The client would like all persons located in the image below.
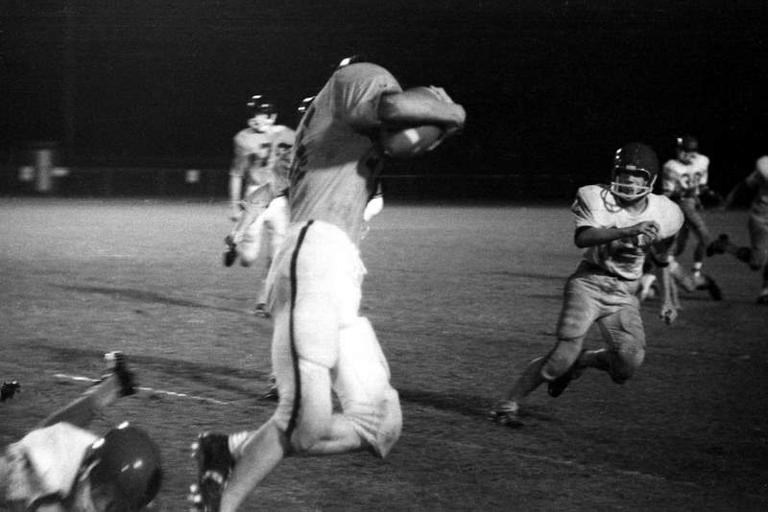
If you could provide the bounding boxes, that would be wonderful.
[641,134,724,307]
[0,349,165,512]
[221,94,299,316]
[490,143,686,429]
[188,53,467,511]
[704,149,767,305]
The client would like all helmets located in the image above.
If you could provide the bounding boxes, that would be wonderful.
[607,140,658,209]
[244,92,280,132]
[675,136,697,157]
[88,420,166,511]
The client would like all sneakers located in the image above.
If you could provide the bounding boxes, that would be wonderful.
[224,235,240,267]
[105,348,139,397]
[667,232,732,302]
[183,432,238,512]
[548,349,588,400]
[490,398,523,427]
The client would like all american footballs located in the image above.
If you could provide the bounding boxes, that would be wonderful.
[380,85,447,158]
